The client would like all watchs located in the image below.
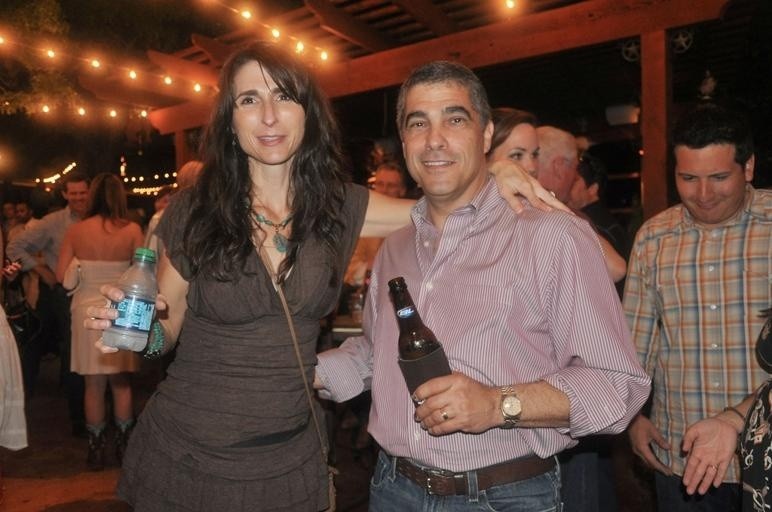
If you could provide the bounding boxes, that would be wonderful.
[138,321,162,361]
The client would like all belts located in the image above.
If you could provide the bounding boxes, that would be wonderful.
[380,446,556,498]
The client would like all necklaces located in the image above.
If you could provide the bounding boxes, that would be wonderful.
[245,204,293,252]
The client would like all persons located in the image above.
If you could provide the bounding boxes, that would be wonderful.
[619,105,772,512]
[313,57,650,512]
[1,159,205,470]
[85,41,577,512]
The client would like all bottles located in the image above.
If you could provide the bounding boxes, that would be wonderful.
[350,268,371,311]
[387,277,451,410]
[101,246,158,354]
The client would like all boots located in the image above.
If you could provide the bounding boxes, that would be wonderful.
[83,421,110,470]
[113,418,136,462]
